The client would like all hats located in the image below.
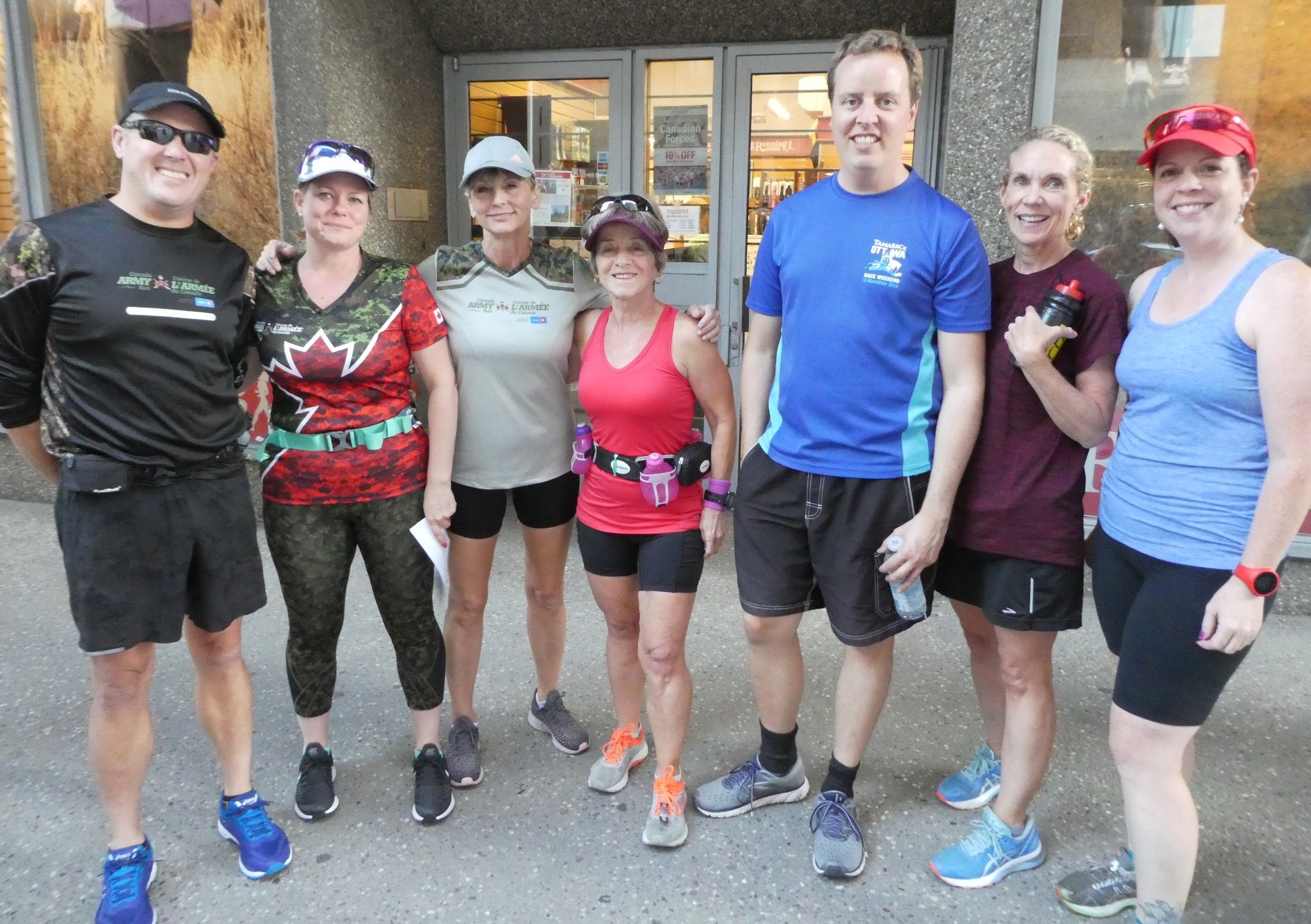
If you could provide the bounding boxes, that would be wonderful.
[119,81,227,139]
[459,136,536,187]
[585,208,663,253]
[298,143,378,192]
[1137,103,1256,178]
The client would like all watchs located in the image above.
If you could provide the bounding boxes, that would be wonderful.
[1229,560,1280,598]
[700,488,737,512]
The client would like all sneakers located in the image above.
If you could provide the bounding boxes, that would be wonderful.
[294,742,339,820]
[411,743,455,823]
[95,833,158,924]
[528,687,590,754]
[929,806,1045,889]
[810,787,868,877]
[587,720,648,792]
[217,786,292,879]
[935,744,1002,810]
[445,716,483,786]
[691,753,809,817]
[642,766,688,846]
[1056,845,1136,917]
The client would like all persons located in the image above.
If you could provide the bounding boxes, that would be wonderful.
[683,31,991,886]
[927,125,1131,894]
[231,137,456,826]
[0,80,295,924]
[1047,103,1310,924]
[73,0,193,128]
[562,195,734,846]
[250,132,724,790]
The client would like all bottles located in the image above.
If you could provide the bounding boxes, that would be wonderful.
[570,422,592,475]
[689,428,702,443]
[640,453,680,505]
[1008,279,1084,369]
[883,536,927,620]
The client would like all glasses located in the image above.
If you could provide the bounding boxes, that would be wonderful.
[584,195,658,222]
[1144,106,1258,155]
[117,118,220,155]
[299,141,375,181]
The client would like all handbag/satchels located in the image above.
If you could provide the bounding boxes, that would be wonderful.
[674,442,712,486]
